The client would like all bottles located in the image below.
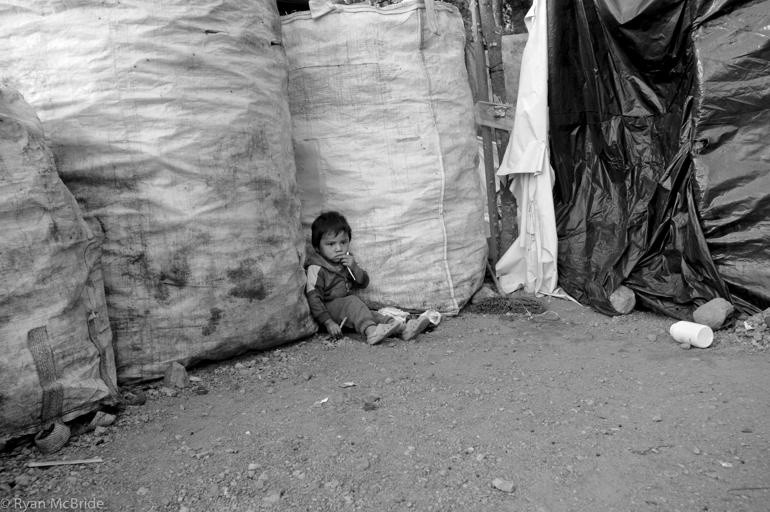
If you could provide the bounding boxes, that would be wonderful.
[670,320,714,349]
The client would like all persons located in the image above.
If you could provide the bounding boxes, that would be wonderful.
[301,211,432,347]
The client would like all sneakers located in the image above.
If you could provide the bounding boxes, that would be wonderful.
[401,316,430,342]
[366,321,400,345]
